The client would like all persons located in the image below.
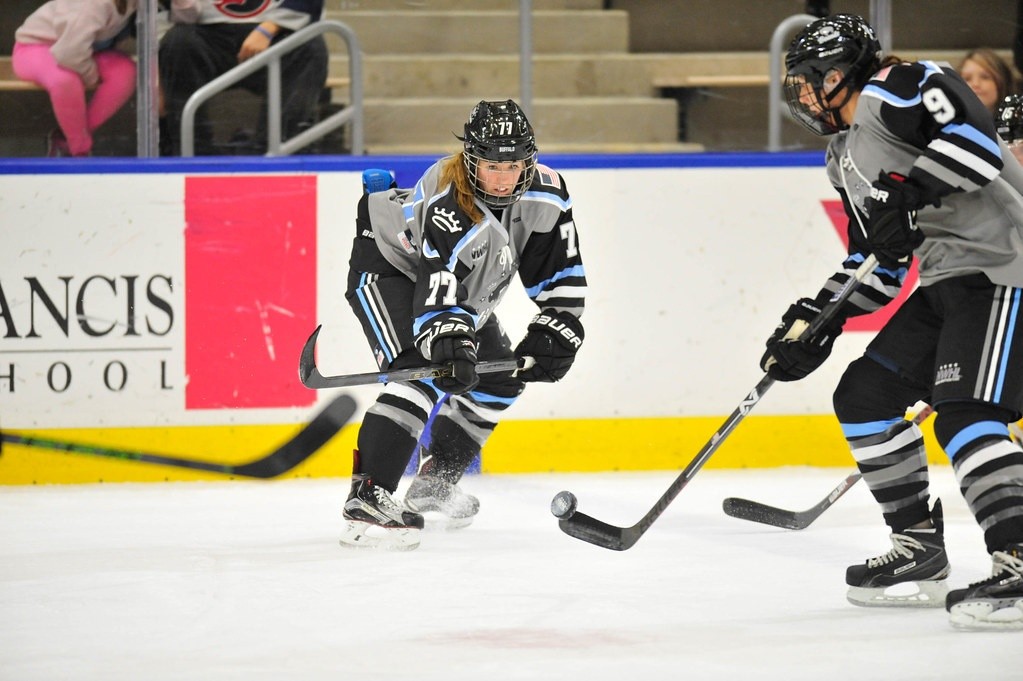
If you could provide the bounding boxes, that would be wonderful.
[994,94,1023,144]
[12,0,137,157]
[759,14,1023,632]
[338,100,587,552]
[158,0,328,156]
[957,49,1012,127]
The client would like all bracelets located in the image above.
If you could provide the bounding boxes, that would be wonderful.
[256,25,272,40]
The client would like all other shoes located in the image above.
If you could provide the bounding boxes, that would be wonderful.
[78,148,93,157]
[47,128,71,157]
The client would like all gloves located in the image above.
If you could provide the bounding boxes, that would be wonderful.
[513,308,585,382]
[759,298,846,381]
[866,166,926,271]
[430,334,480,396]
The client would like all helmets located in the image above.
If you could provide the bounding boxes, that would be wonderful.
[782,13,882,137]
[462,99,538,210]
[994,93,1023,147]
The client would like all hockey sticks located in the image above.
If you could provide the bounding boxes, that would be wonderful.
[559,252,880,552]
[3,392,357,480]
[299,325,538,390]
[722,405,935,531]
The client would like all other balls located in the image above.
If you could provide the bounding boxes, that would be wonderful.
[551,490,578,521]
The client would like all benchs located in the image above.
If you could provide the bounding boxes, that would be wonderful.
[0,53,352,152]
[647,49,1023,143]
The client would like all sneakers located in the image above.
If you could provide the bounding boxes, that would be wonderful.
[946,542,1023,632]
[846,497,951,604]
[403,478,480,530]
[339,447,424,546]
